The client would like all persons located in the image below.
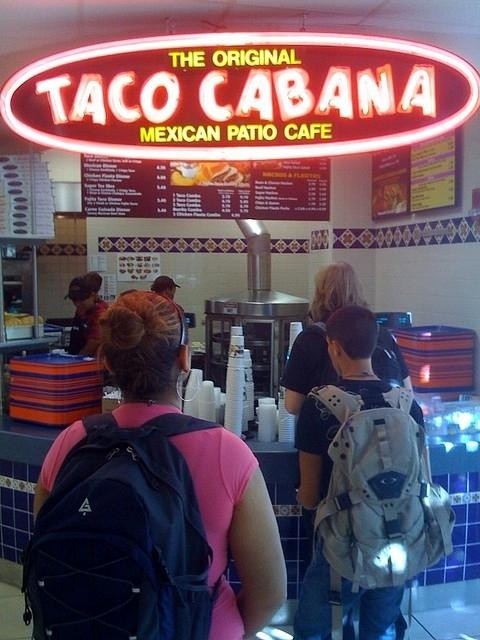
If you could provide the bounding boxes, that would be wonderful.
[63,271,111,356]
[33,291,287,640]
[151,274,181,301]
[277,261,413,529]
[290,305,427,640]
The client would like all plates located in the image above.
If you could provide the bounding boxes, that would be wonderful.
[394,325,477,392]
[8,354,104,429]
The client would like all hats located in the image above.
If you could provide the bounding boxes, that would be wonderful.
[151,276,181,291]
[64,277,94,301]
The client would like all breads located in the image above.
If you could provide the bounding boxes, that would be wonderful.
[3,311,43,327]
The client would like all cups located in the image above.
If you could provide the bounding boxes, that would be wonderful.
[278,382,296,444]
[288,320,302,357]
[182,321,254,444]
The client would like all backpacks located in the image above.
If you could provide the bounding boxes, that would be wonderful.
[308,384,456,593]
[22,411,228,639]
